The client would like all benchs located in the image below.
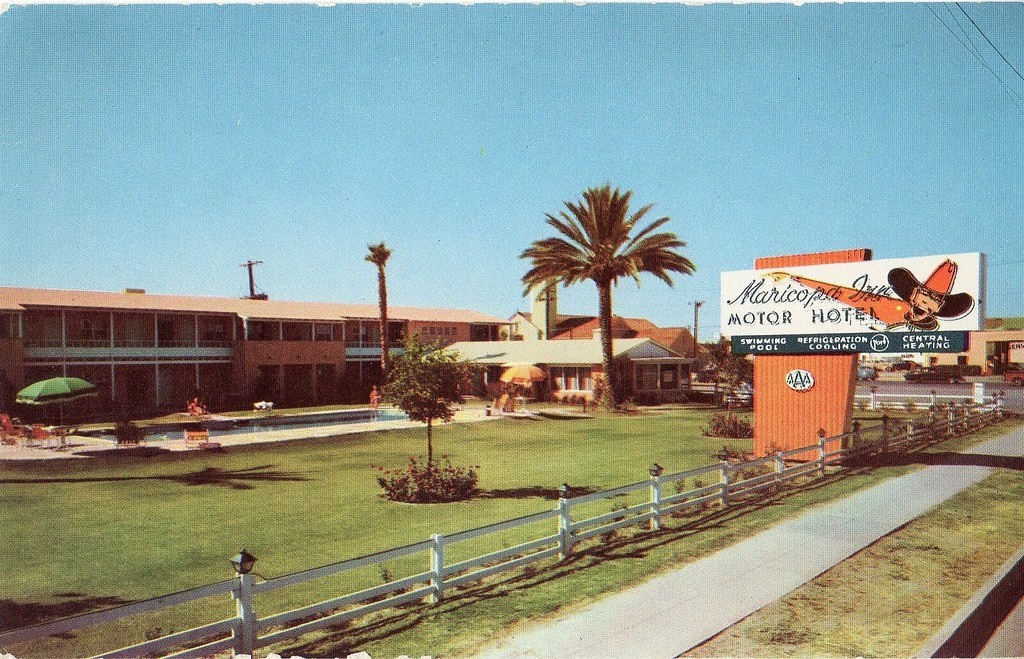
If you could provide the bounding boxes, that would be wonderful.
[183,429,210,449]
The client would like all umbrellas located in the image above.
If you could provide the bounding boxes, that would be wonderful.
[500,364,549,400]
[16,375,98,449]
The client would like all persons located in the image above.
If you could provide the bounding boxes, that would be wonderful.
[188,397,212,420]
[368,384,383,424]
[495,389,522,416]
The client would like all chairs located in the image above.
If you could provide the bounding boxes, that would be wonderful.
[1,413,51,449]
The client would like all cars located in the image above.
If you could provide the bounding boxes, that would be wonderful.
[856,359,1024,387]
